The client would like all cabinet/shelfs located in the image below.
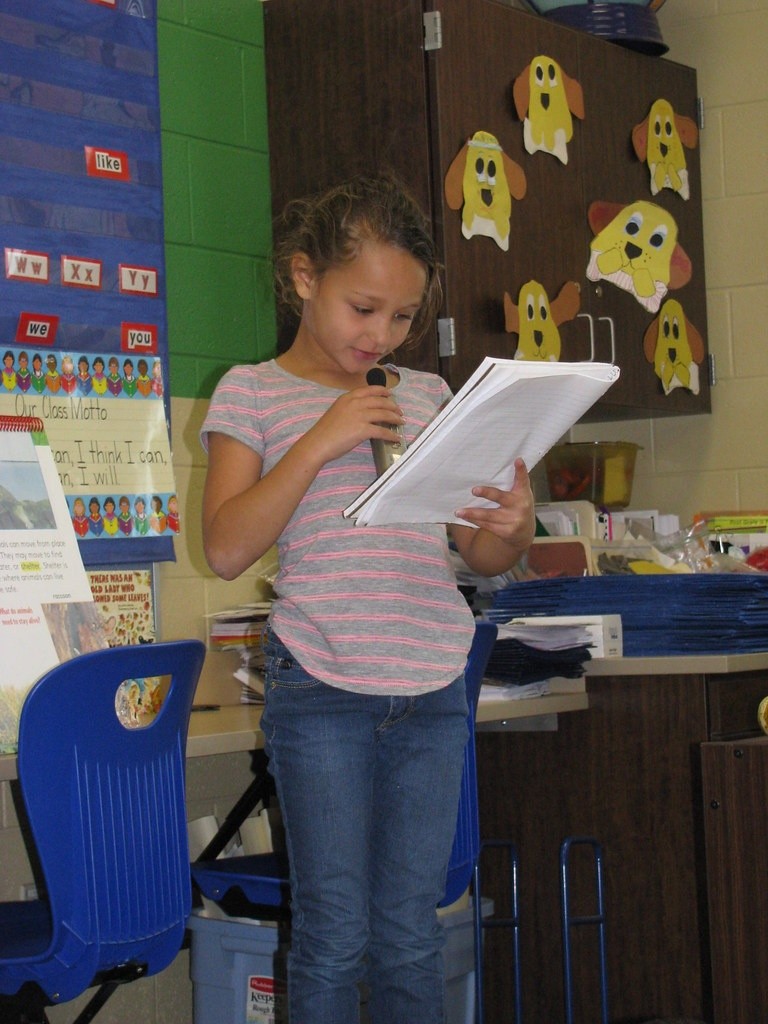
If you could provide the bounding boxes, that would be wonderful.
[263,0,715,425]
[475,653,768,1024]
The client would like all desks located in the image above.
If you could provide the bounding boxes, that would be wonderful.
[0,691,591,1024]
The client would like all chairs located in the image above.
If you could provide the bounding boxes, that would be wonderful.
[189,622,499,922]
[0,637,207,1024]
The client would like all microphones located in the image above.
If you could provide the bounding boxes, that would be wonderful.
[366,368,407,477]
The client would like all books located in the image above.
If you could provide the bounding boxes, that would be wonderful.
[208,355,766,706]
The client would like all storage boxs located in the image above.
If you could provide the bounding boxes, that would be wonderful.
[542,440,645,508]
[512,614,622,660]
[184,897,494,1024]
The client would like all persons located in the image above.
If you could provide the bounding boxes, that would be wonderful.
[198,168,540,1024]
